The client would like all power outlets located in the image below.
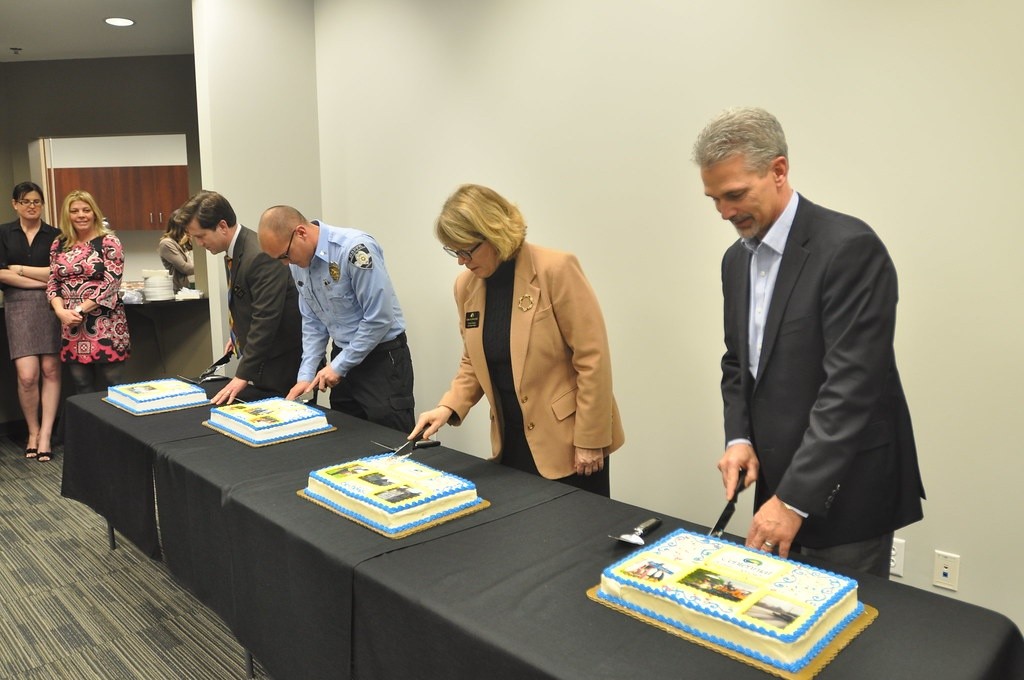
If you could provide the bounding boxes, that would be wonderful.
[889,538,905,578]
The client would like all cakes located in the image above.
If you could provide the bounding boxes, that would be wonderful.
[208,395,334,445]
[106,377,211,414]
[305,452,482,533]
[596,528,864,673]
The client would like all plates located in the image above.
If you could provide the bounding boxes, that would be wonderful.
[144,276,174,301]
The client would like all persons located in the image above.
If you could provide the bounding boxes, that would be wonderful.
[158,209,196,293]
[174,189,327,404]
[46,191,131,394]
[0,182,62,462]
[408,185,625,497]
[694,108,926,579]
[258,206,417,435]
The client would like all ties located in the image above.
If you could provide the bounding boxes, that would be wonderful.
[224,254,243,360]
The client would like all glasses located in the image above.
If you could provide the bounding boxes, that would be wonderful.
[272,230,296,261]
[15,198,44,207]
[442,237,486,261]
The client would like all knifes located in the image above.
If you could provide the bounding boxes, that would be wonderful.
[707,470,746,539]
[389,424,431,459]
[198,350,234,378]
[295,384,319,399]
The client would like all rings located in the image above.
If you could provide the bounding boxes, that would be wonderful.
[764,541,774,549]
[227,391,231,394]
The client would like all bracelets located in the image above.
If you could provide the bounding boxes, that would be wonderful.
[20,265,23,276]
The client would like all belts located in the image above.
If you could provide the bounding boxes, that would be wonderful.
[332,332,407,351]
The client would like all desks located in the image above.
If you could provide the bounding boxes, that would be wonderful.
[57,372,1023,680]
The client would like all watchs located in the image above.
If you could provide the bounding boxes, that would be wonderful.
[74,305,85,315]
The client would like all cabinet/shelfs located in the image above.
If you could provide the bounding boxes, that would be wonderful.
[27,137,189,231]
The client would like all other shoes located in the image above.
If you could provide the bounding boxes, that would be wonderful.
[25,448,52,462]
